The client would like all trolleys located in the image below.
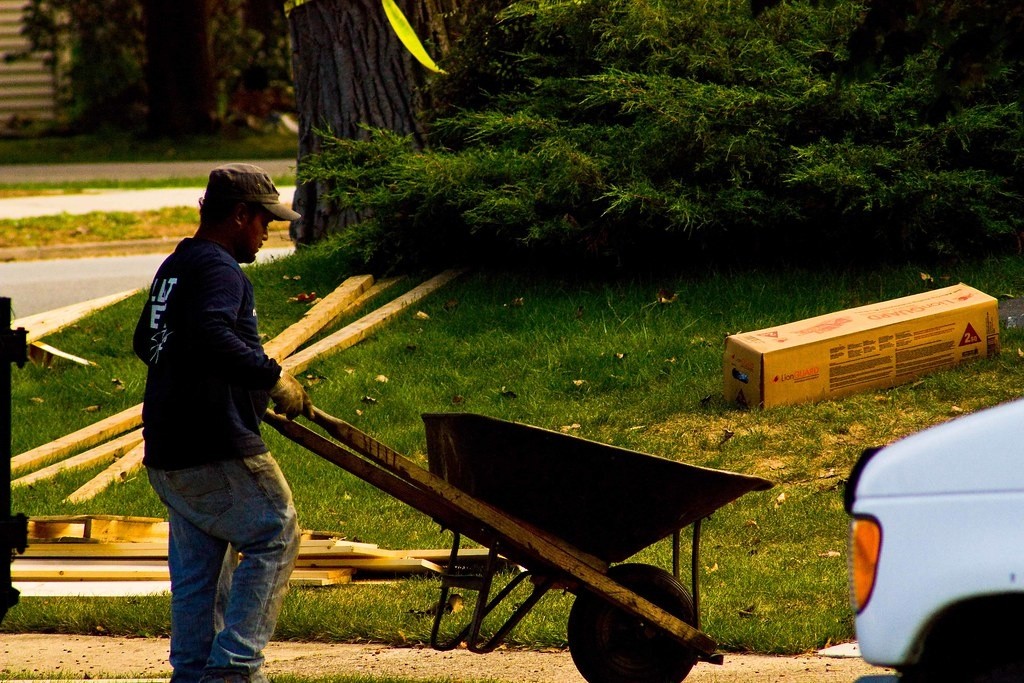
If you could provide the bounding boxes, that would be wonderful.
[261,404,778,683]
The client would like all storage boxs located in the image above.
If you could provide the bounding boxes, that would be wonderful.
[722,281,1001,412]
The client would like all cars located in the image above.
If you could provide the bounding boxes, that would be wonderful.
[842,399,1024,683]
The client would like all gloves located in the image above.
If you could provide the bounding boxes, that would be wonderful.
[269,371,315,421]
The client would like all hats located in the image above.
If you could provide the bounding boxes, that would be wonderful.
[207,161,301,221]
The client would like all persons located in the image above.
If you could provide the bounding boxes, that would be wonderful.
[131,163,315,683]
